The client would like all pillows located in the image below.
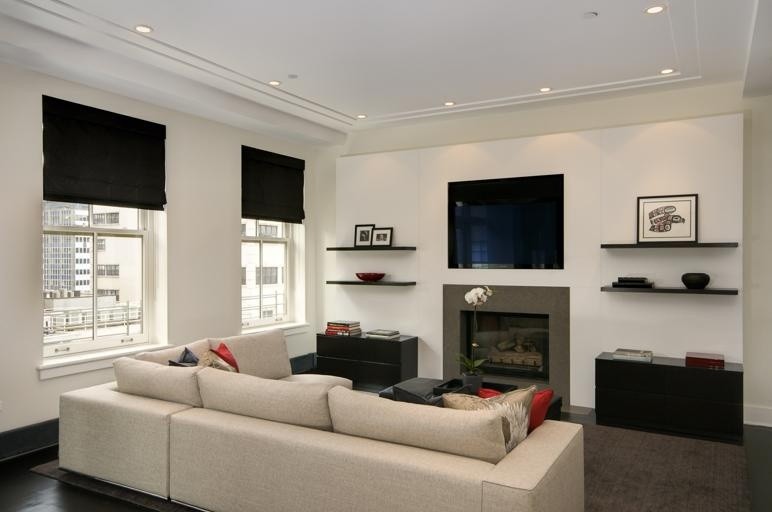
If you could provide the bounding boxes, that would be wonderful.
[195,350,238,373]
[166,345,201,368]
[207,341,240,373]
[392,384,444,408]
[544,393,563,423]
[379,389,392,399]
[477,386,555,434]
[440,383,538,456]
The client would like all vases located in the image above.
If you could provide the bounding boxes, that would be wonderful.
[458,369,484,394]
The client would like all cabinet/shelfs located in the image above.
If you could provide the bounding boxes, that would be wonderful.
[326,245,416,286]
[599,242,741,295]
[593,350,746,448]
[313,328,419,383]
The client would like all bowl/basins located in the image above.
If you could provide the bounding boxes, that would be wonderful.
[681,272,710,289]
[355,272,385,281]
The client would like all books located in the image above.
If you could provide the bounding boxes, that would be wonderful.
[612,348,653,362]
[612,277,655,288]
[325,320,402,340]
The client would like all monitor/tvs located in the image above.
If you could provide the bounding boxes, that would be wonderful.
[448,174,564,269]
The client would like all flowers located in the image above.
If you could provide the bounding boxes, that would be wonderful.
[450,284,497,377]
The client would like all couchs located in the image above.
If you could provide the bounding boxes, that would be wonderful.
[53,326,590,512]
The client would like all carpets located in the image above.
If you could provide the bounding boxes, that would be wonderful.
[30,409,755,512]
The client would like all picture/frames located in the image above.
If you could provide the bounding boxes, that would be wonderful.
[637,194,698,241]
[354,224,375,247]
[370,227,393,248]
